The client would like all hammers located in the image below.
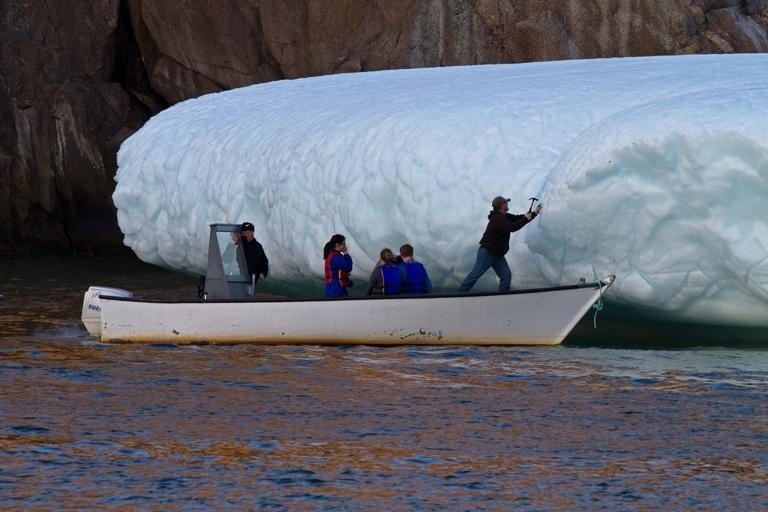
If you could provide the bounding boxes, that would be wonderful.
[527,197,539,213]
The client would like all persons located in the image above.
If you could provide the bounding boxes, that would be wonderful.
[324,235,352,297]
[222,231,248,275]
[400,244,432,294]
[241,223,268,285]
[458,195,542,292]
[364,249,409,297]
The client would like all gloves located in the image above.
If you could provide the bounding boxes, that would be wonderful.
[524,212,532,221]
[533,203,542,216]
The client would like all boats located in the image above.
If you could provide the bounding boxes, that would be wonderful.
[81,274,618,347]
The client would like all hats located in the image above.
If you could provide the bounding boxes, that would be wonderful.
[492,196,510,209]
[241,222,254,231]
